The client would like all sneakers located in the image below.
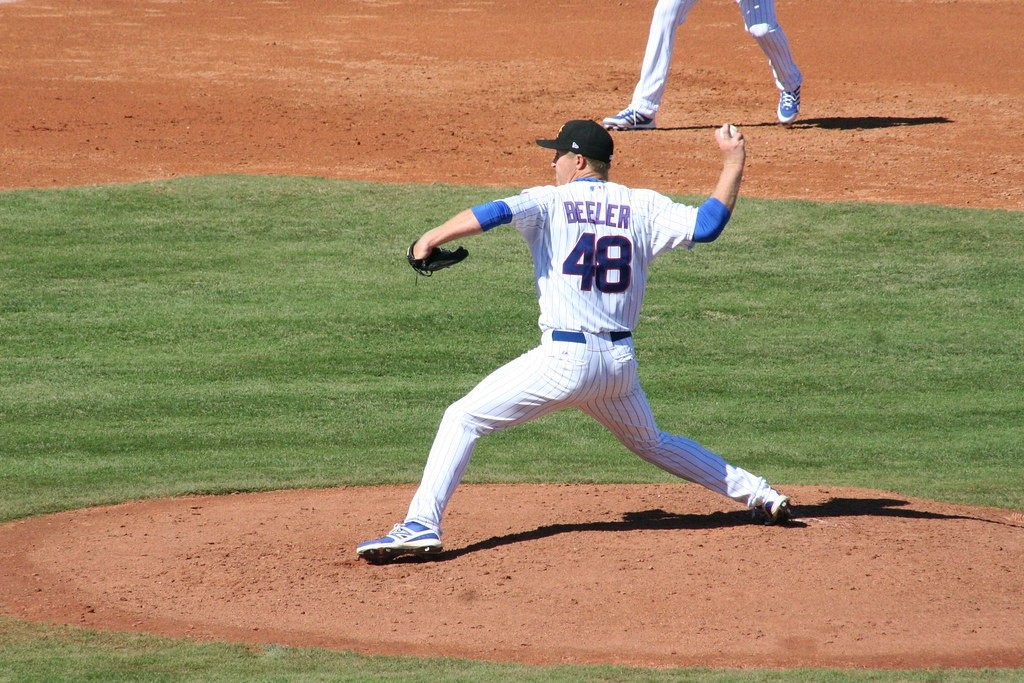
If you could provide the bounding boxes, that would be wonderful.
[603,107,656,130]
[356,522,442,563]
[752,488,791,524]
[777,85,801,125]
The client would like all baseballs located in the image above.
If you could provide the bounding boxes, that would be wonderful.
[719,124,738,138]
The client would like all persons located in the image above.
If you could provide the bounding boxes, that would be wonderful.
[356,120,795,562]
[603,0,803,129]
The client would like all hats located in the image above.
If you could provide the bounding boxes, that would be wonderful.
[536,119,614,164]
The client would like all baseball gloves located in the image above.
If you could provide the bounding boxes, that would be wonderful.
[405,240,469,272]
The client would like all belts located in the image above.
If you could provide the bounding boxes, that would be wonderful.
[552,330,632,344]
